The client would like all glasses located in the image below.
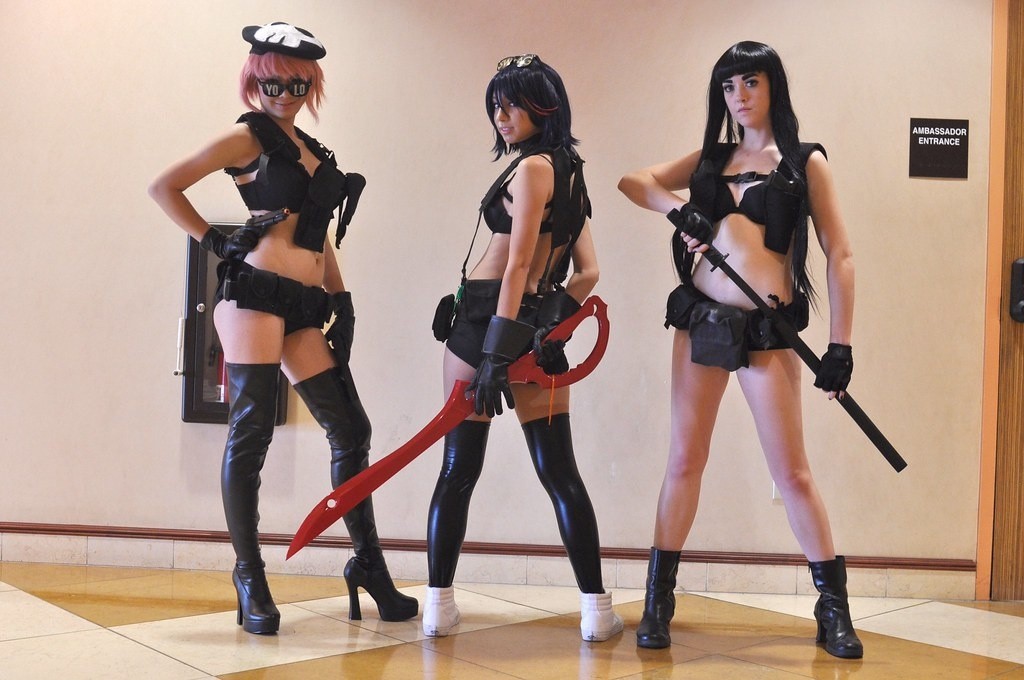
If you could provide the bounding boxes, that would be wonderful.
[496,53,536,71]
[256,78,312,97]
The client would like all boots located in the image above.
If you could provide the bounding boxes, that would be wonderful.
[291,365,419,621]
[635,547,682,648]
[807,554,863,658]
[220,363,281,634]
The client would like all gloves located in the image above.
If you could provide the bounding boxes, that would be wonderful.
[198,226,259,259]
[533,289,583,375]
[464,313,538,419]
[323,291,355,371]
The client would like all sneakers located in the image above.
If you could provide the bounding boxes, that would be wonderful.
[421,584,462,637]
[580,590,624,642]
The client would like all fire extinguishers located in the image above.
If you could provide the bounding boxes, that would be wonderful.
[207,340,229,404]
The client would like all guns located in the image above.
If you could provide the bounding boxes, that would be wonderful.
[765,169,805,199]
[234,207,290,260]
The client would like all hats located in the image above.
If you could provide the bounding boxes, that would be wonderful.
[241,22,327,60]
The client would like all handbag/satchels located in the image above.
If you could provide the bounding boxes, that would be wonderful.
[688,299,748,372]
[464,279,502,324]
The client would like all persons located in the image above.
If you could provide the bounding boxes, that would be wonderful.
[618,41,865,659]
[147,21,419,633]
[423,57,624,643]
